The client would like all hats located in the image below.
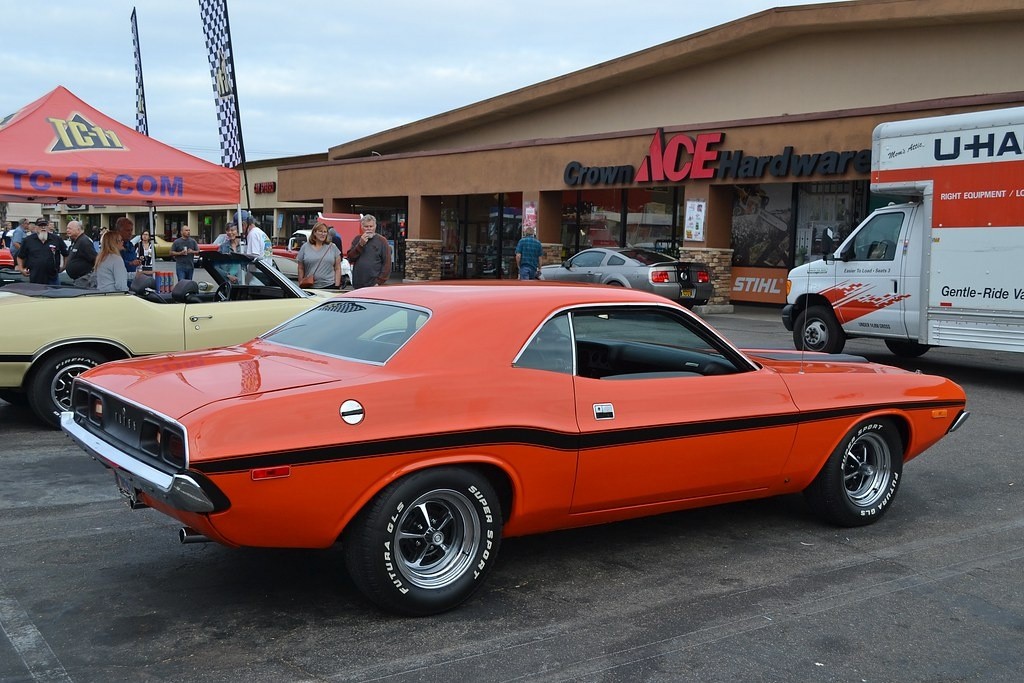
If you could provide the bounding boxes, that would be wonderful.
[231,211,251,228]
[37,218,48,226]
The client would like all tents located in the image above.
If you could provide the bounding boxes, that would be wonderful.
[0,84,245,254]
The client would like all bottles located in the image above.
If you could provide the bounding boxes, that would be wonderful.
[153,270,174,293]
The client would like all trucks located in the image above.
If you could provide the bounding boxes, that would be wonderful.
[780,106,1024,380]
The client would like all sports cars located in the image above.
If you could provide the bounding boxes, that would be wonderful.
[0,249,359,431]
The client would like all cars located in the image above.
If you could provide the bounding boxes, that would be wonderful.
[55,276,975,608]
[1,244,75,286]
[537,244,717,314]
[188,232,355,288]
[128,233,181,262]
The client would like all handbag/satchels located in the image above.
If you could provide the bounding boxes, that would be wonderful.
[299,275,315,289]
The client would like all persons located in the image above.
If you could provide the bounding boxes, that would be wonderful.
[91,225,111,247]
[229,210,274,288]
[0,218,61,271]
[326,225,343,263]
[346,213,392,290]
[515,227,543,281]
[88,231,130,293]
[169,225,199,282]
[14,217,69,285]
[115,217,144,279]
[134,229,154,278]
[213,223,243,286]
[64,221,98,289]
[294,222,343,289]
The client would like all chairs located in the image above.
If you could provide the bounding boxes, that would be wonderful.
[130,270,202,304]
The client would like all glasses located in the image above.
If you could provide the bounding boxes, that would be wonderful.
[116,239,123,243]
